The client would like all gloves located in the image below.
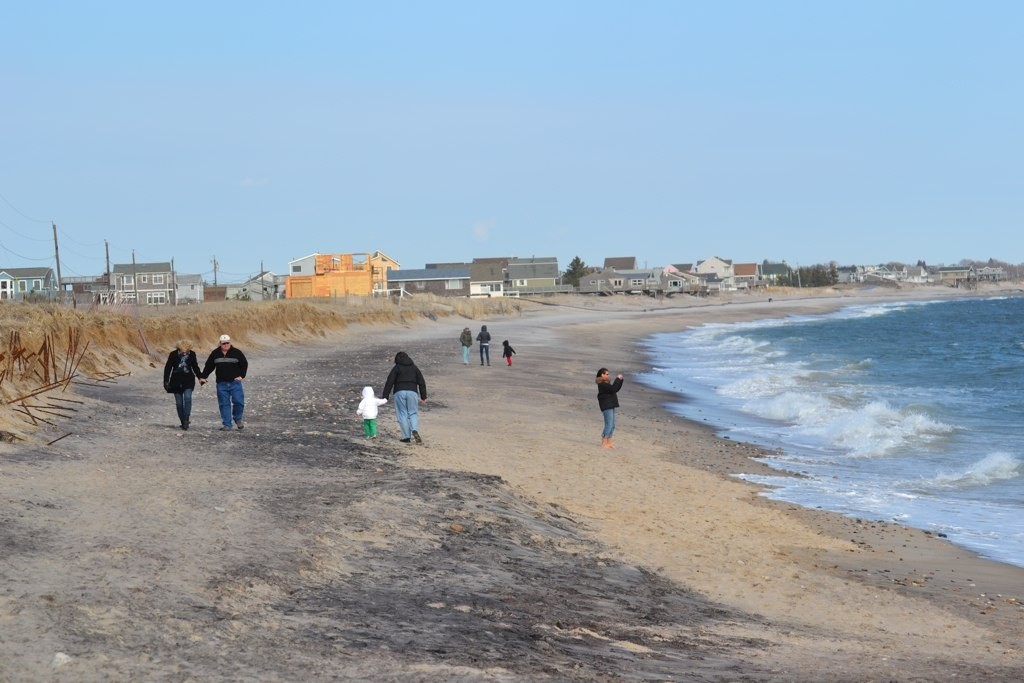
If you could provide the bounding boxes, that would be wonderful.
[164,381,169,391]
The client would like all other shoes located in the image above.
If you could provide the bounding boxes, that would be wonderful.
[237,422,244,429]
[181,421,190,430]
[412,429,421,443]
[221,425,231,431]
[371,434,376,439]
[366,436,370,440]
[400,438,410,442]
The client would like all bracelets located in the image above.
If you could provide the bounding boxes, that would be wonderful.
[239,376,242,380]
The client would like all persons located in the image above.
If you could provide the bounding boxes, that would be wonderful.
[475,325,491,366]
[594,367,624,449]
[502,339,516,366]
[355,386,388,439]
[163,339,208,430]
[201,334,249,430]
[382,351,427,444]
[460,326,473,365]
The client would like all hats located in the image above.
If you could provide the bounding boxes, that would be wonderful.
[219,335,230,343]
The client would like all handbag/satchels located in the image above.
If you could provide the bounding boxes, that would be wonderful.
[168,375,187,393]
